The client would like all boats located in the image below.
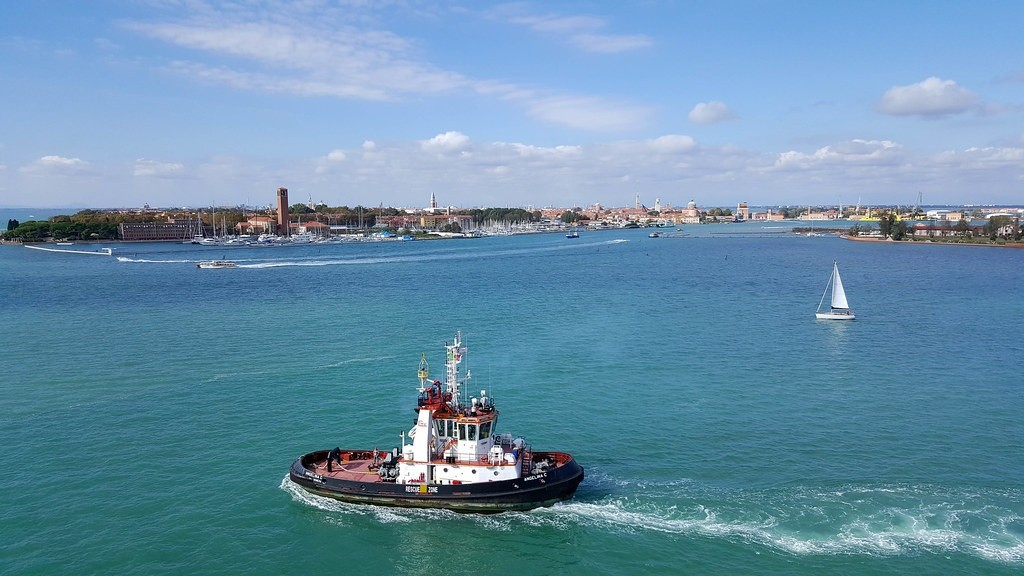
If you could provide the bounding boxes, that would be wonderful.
[284,328,587,518]
[183,205,689,240]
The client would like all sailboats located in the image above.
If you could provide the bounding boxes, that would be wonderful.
[814,260,855,320]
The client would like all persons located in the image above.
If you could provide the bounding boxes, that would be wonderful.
[373,447,380,463]
